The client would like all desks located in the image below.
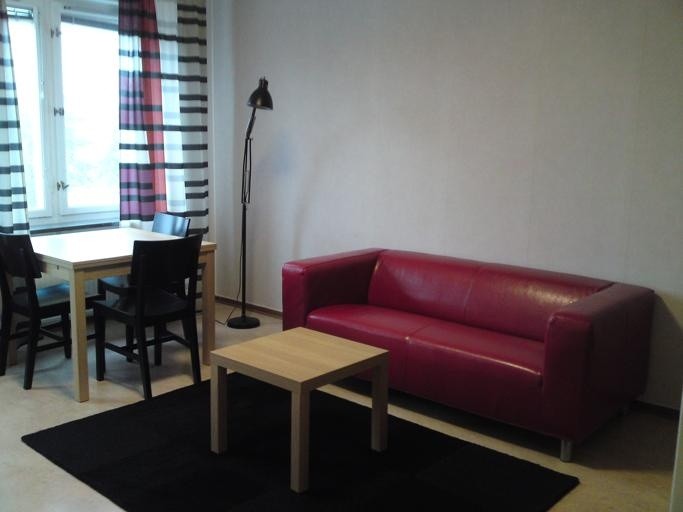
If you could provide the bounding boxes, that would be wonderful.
[202,324,390,495]
[25,233,217,403]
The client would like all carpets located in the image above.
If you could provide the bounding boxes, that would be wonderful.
[22,369,579,512]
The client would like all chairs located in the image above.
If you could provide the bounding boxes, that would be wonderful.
[92,210,193,368]
[91,226,212,400]
[0,230,107,390]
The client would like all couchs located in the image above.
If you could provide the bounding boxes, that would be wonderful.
[278,244,653,459]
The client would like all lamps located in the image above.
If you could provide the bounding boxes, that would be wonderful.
[227,74,275,328]
